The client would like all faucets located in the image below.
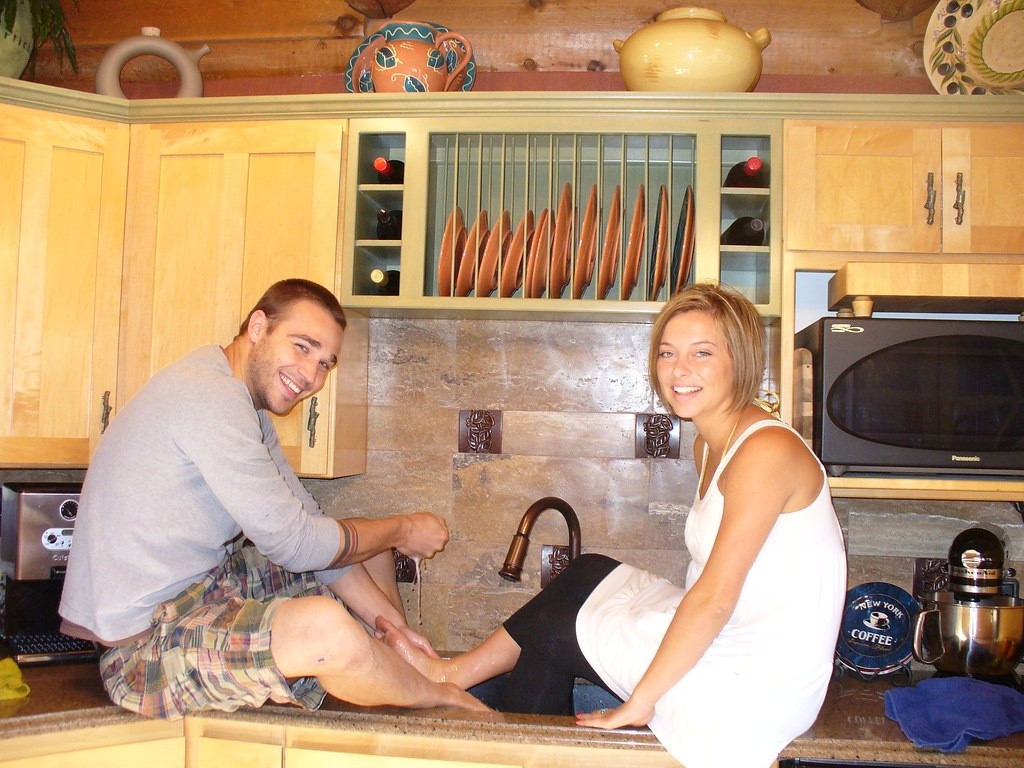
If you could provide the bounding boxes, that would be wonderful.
[499,496,581,583]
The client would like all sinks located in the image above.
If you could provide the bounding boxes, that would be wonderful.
[299,661,671,726]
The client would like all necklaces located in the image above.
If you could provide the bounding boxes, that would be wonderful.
[698,407,744,498]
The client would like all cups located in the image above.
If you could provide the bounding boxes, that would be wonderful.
[870,612,889,626]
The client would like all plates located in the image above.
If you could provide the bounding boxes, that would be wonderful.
[622,184,645,301]
[650,185,667,301]
[834,582,921,675]
[438,207,467,296]
[923,0,1024,95]
[478,210,513,297]
[500,210,535,298]
[863,619,890,630]
[550,184,578,299]
[345,21,476,93]
[597,185,626,300]
[457,210,490,297]
[670,185,695,299]
[574,184,602,300]
[525,208,547,299]
[531,209,556,299]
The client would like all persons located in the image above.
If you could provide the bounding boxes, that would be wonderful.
[374,281,846,767]
[60,279,504,713]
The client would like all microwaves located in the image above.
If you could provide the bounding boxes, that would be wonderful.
[794,317,1024,477]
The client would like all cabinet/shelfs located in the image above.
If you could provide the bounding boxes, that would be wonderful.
[1,76,1024,502]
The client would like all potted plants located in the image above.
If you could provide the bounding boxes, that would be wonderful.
[0,0,82,79]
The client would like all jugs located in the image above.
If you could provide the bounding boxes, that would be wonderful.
[372,20,472,92]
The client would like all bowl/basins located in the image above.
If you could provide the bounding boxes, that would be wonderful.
[911,591,1024,678]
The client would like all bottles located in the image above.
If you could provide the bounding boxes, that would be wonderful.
[723,157,764,188]
[374,157,404,184]
[721,217,764,246]
[377,208,402,240]
[370,269,400,296]
